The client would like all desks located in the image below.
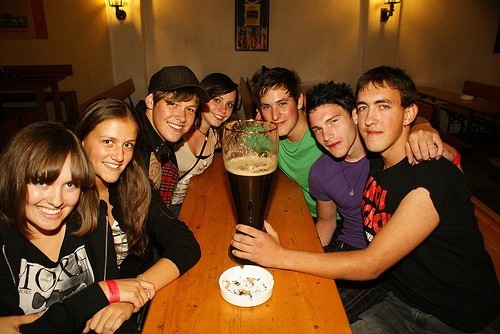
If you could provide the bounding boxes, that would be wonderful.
[416,86,500,140]
[139,153,352,334]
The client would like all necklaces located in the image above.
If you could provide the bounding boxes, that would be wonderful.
[340,157,367,196]
[198,129,208,141]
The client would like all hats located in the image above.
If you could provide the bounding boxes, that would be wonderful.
[148,66,210,104]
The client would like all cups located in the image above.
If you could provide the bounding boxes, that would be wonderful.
[222,118,279,267]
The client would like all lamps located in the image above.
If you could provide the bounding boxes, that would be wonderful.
[381,0,400,23]
[108,0,127,20]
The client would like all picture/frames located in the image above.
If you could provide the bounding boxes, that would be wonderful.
[235,0,270,51]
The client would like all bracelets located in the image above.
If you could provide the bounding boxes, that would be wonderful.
[104,279,120,303]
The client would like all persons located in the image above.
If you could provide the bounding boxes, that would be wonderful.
[0,99,201,334]
[133,65,210,213]
[230,66,500,334]
[251,66,343,252]
[172,73,239,219]
[307,80,444,301]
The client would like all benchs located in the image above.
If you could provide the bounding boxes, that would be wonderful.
[446,80,500,135]
[416,98,461,148]
